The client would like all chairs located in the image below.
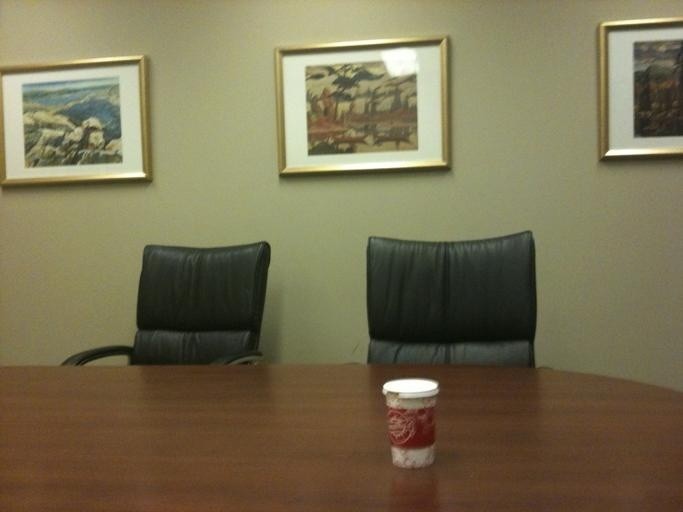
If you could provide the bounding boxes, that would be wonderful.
[360,230,536,369]
[63,243,276,368]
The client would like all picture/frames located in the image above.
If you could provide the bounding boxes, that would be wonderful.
[273,35,451,178]
[0,55,150,187]
[594,15,683,162]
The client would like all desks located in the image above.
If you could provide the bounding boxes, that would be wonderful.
[0,360,681,512]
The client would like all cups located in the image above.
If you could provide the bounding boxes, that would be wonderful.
[381,377,440,469]
